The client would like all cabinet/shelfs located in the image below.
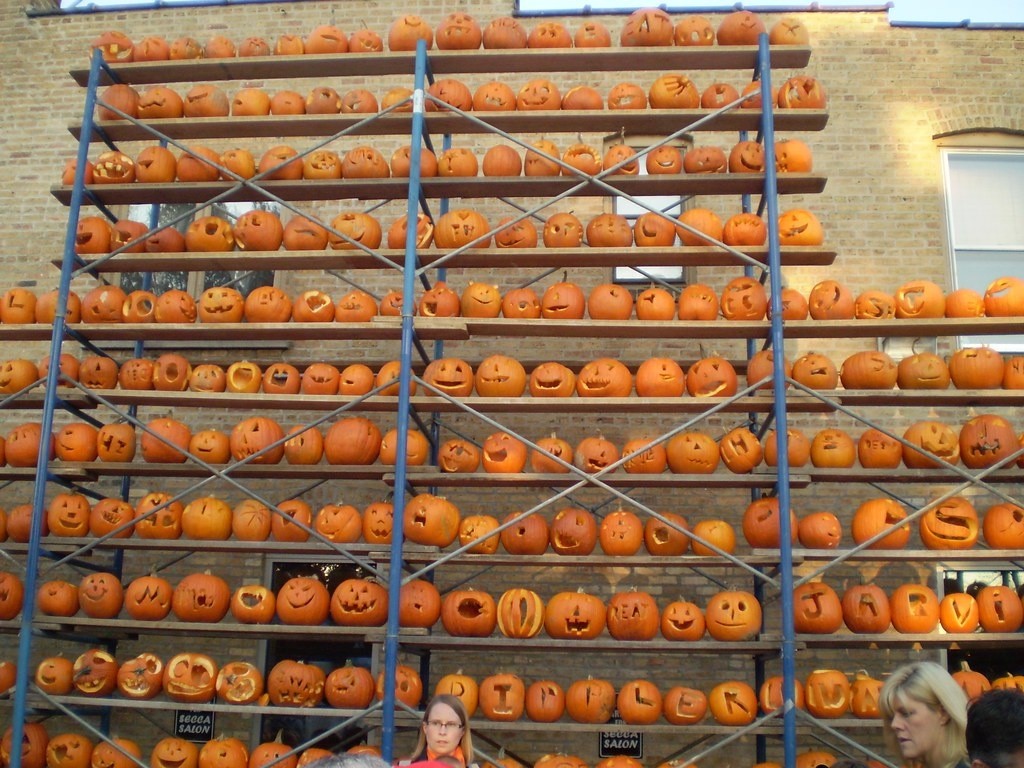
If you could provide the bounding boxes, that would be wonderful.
[0,32,1024,768]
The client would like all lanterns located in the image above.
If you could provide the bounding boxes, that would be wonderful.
[55,128,819,187]
[0,415,1023,472]
[1,648,1024,724]
[868,757,925,767]
[67,205,825,255]
[1,494,1023,556]
[80,6,810,60]
[485,747,838,768]
[0,343,1024,398]
[0,573,1024,642]
[97,72,827,121]
[1,274,1023,324]
[0,719,382,766]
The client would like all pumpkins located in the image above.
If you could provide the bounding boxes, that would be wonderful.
[482,144,522,178]
[98,84,139,121]
[676,208,723,246]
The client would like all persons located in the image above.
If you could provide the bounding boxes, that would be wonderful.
[964,686,1024,768]
[295,754,394,768]
[878,661,971,767]
[397,695,483,768]
[435,756,463,768]
[827,759,870,768]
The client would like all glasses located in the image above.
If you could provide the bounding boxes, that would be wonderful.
[428,720,465,729]
